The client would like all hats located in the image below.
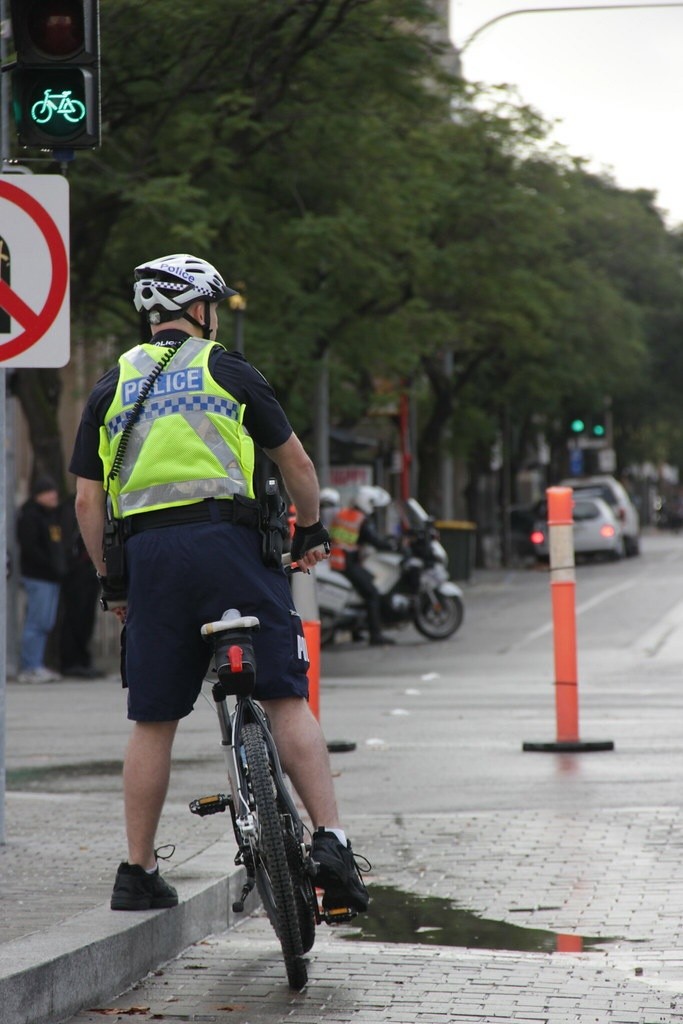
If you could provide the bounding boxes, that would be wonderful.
[30,472,57,494]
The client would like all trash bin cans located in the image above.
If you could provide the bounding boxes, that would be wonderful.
[434,520,479,580]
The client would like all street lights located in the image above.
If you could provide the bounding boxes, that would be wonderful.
[229,281,247,355]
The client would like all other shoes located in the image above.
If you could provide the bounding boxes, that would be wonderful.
[17,666,61,682]
[368,630,397,645]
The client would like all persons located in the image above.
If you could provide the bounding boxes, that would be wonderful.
[66,253,371,914]
[14,473,109,684]
[327,484,397,647]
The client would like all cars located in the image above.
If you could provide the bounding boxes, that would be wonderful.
[530,496,626,563]
[559,474,640,556]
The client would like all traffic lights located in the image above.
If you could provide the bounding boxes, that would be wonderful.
[588,418,606,438]
[568,416,588,435]
[7,0,102,151]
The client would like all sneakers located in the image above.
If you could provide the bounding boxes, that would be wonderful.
[110,848,179,910]
[309,826,369,913]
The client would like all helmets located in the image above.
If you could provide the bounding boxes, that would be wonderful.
[133,253,238,325]
[354,484,392,515]
[319,487,341,506]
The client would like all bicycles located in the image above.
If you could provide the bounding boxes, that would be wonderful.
[99,541,359,992]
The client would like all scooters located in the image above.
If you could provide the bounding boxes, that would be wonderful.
[314,498,464,650]
